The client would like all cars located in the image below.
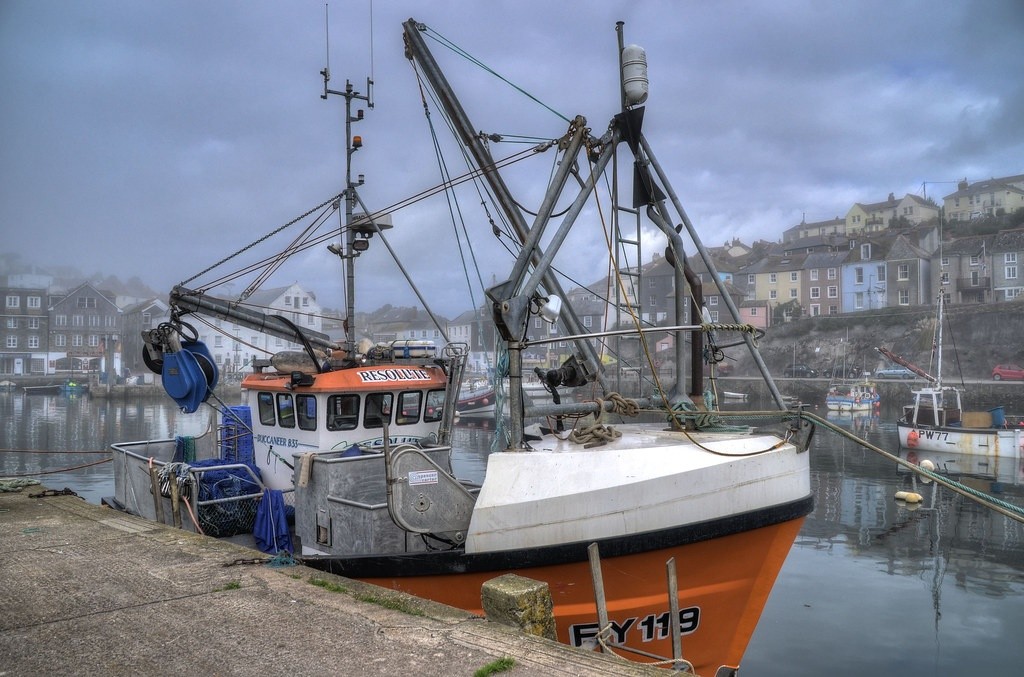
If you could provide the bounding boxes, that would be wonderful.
[822,364,861,380]
[874,363,914,380]
[990,360,1022,381]
[784,363,817,378]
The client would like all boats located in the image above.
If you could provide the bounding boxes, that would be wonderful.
[894,274,1023,457]
[825,323,880,411]
[104,0,816,671]
